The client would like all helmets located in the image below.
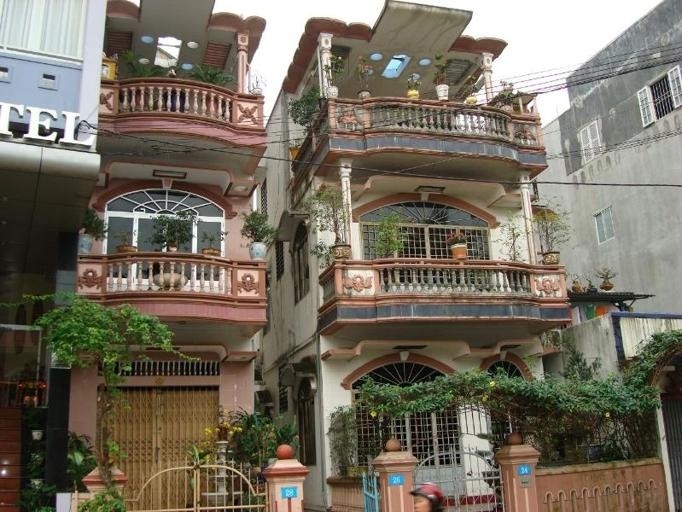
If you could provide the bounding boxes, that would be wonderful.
[407,484,444,507]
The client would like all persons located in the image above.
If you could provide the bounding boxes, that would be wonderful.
[409,484,445,512]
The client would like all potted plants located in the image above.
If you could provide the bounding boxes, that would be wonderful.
[370,203,416,292]
[19,405,51,490]
[323,51,517,115]
[76,203,280,262]
[305,183,353,259]
[120,51,238,121]
[570,265,617,293]
[492,188,573,292]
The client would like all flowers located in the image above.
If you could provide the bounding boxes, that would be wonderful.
[445,231,467,245]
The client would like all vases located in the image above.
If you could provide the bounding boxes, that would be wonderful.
[450,244,467,260]
[289,146,299,159]
[577,441,604,464]
